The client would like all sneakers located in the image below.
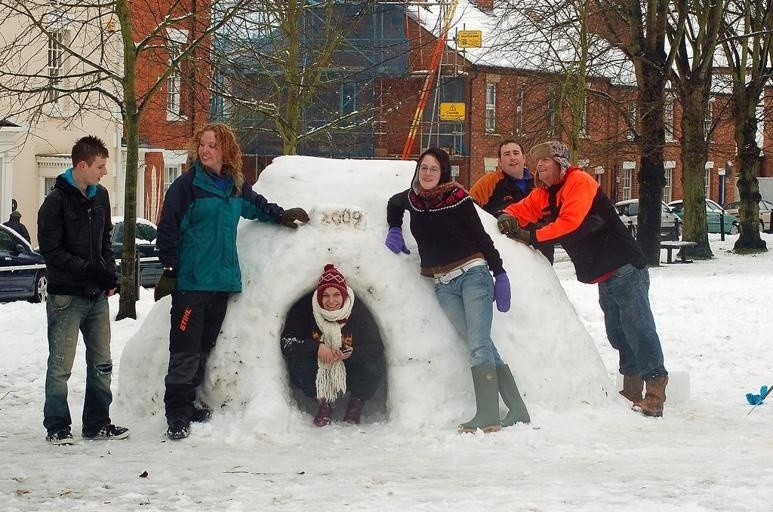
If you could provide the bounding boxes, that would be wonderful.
[82,426,130,439]
[166,424,189,438]
[46,433,72,444]
[191,408,210,422]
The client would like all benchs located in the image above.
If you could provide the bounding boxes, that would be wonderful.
[628,217,698,263]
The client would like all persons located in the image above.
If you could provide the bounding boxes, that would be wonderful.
[469,139,554,265]
[494,141,668,417]
[37,134,129,444]
[154,122,309,439]
[385,148,530,434]
[2,211,31,244]
[281,264,384,428]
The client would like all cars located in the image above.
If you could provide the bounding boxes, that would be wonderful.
[667,199,740,235]
[614,198,683,241]
[32,216,163,296]
[722,200,773,234]
[0,223,48,304]
[611,205,637,239]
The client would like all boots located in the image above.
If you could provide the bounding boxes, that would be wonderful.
[458,364,499,432]
[632,375,668,416]
[315,396,332,426]
[497,364,529,426]
[619,372,644,402]
[345,397,364,423]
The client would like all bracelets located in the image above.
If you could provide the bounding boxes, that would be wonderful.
[163,267,174,272]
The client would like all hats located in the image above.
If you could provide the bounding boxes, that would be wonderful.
[530,142,570,187]
[317,264,347,309]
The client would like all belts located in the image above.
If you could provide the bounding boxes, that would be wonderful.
[433,260,488,285]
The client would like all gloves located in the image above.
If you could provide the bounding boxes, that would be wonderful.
[492,273,511,311]
[386,226,410,255]
[86,266,118,290]
[153,271,176,301]
[281,208,308,228]
[498,213,518,234]
[510,229,531,245]
